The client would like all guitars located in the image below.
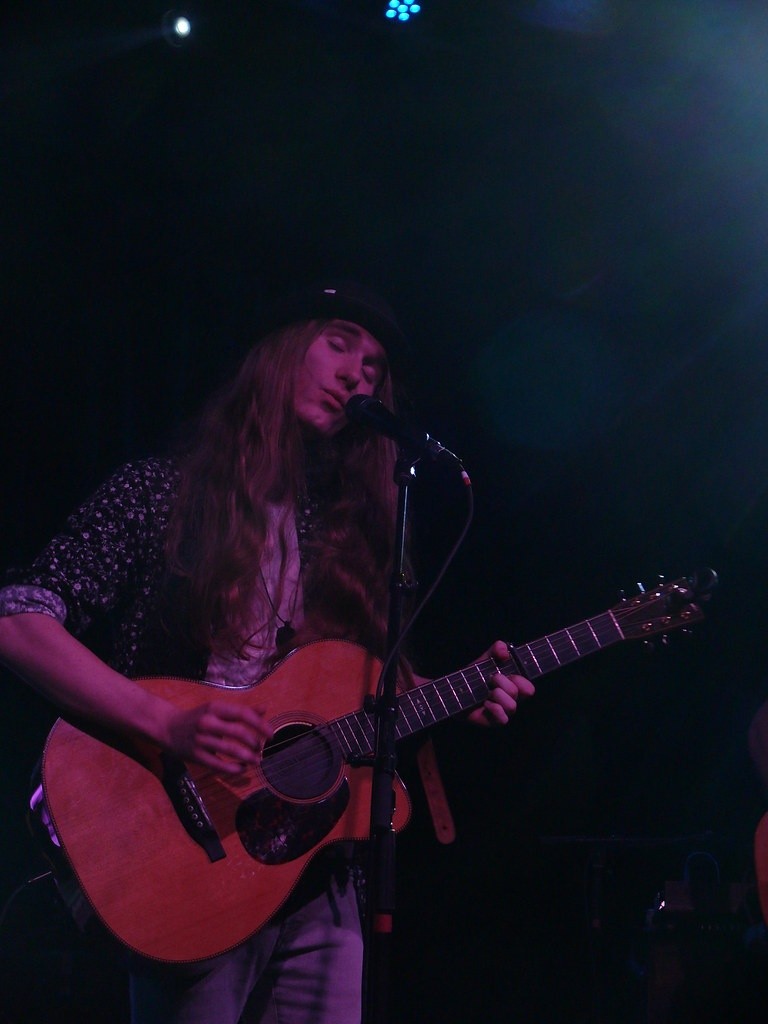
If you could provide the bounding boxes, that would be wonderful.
[27,568,717,992]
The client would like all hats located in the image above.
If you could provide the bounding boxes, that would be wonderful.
[266,278,414,374]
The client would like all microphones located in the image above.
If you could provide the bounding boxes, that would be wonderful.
[346,394,463,467]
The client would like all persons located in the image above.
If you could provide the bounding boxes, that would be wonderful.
[0,259,537,1024]
